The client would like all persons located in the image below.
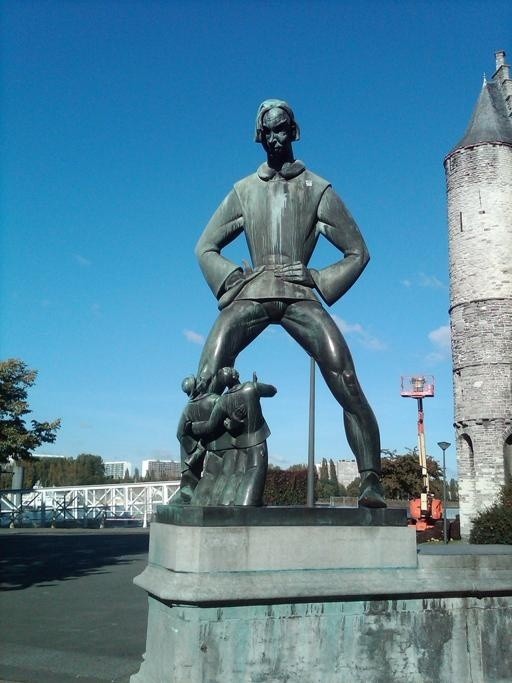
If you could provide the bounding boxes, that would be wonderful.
[194,97,385,507]
[171,366,277,506]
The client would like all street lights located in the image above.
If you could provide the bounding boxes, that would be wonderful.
[437,442,451,544]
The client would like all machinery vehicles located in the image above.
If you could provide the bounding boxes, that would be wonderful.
[400,375,450,531]
